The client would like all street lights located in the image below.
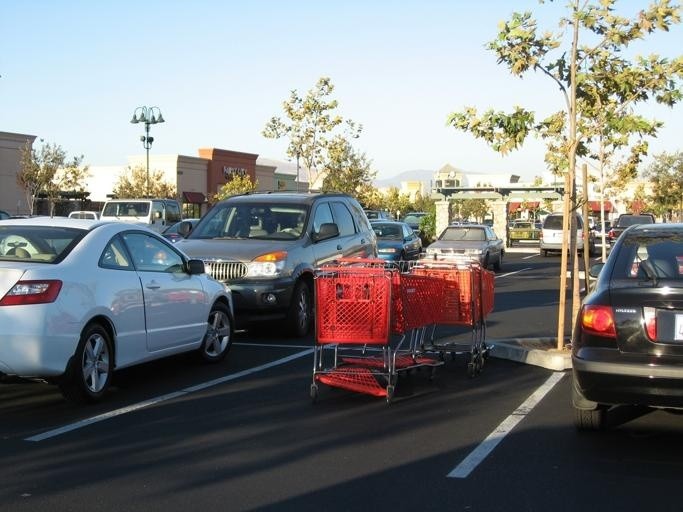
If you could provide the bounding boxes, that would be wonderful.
[130,106,166,198]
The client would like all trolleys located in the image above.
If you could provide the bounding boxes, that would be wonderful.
[430,254,495,360]
[340,261,445,381]
[318,258,461,386]
[417,259,484,369]
[406,265,481,381]
[310,267,402,407]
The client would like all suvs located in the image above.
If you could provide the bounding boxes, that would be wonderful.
[609,214,656,249]
[173,195,376,337]
[539,212,596,256]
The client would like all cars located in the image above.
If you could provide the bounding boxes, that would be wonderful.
[573,224,683,429]
[426,225,504,270]
[511,223,539,241]
[0,215,233,402]
[371,222,421,267]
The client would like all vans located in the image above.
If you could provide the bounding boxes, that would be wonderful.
[68,212,99,219]
[100,200,181,224]
